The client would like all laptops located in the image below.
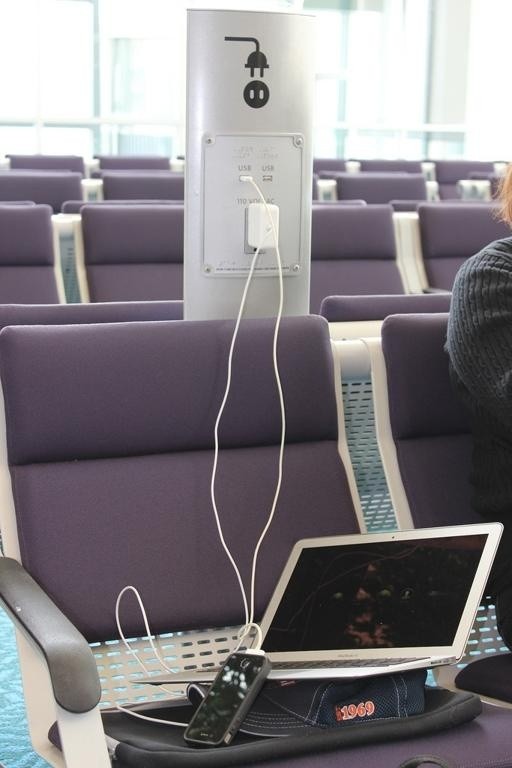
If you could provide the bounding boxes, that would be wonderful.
[130,522,505,685]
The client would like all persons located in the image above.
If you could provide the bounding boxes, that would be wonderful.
[447,163,512,651]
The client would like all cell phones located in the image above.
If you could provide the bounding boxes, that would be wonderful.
[184,652,272,748]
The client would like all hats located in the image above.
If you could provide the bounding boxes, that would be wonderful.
[187,668,431,739]
